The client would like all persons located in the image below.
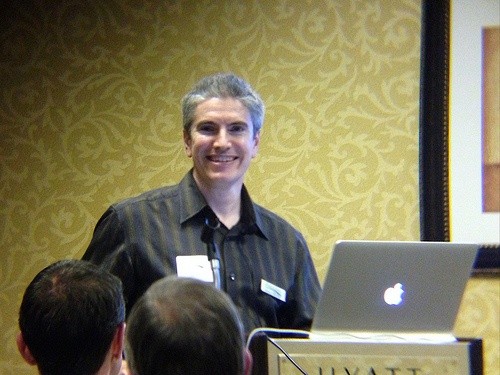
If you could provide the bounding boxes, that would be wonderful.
[79,71,322,375]
[15,258,125,375]
[126,274,253,374]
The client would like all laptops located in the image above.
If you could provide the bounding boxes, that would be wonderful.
[308,241,479,341]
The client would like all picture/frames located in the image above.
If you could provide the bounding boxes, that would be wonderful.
[419,0,500,276]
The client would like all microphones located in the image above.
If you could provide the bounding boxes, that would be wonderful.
[198,216,222,260]
